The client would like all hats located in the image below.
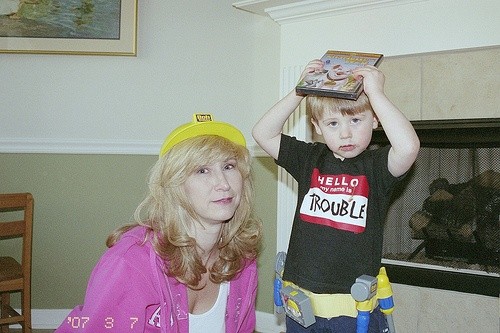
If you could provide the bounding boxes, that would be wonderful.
[159,113,246,159]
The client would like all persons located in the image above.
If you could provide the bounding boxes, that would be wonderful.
[251,58,420,333]
[55,113,263,333]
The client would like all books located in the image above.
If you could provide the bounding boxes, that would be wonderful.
[296,50,383,101]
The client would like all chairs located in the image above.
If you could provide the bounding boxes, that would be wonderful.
[0,193,34,333]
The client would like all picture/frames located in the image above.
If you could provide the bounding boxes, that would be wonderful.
[0,0,138,58]
